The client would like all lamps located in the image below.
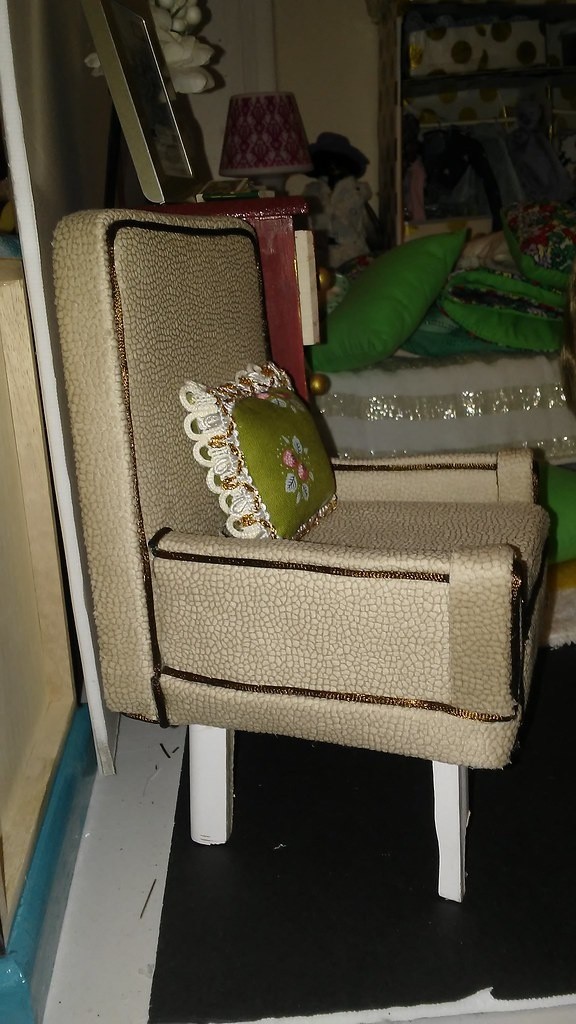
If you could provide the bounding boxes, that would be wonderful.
[218,91,313,192]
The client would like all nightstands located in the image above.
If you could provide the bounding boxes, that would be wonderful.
[119,197,335,409]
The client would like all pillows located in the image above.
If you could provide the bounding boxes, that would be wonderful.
[438,270,564,352]
[399,306,524,358]
[500,205,576,288]
[307,227,473,372]
[453,231,520,276]
[179,361,338,539]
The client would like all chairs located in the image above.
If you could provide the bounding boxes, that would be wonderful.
[51,206,556,902]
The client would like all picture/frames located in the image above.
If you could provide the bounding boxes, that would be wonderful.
[79,0,202,204]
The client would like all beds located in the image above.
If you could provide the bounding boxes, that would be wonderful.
[306,202,576,568]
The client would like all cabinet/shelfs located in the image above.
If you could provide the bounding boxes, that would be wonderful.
[365,0,576,247]
